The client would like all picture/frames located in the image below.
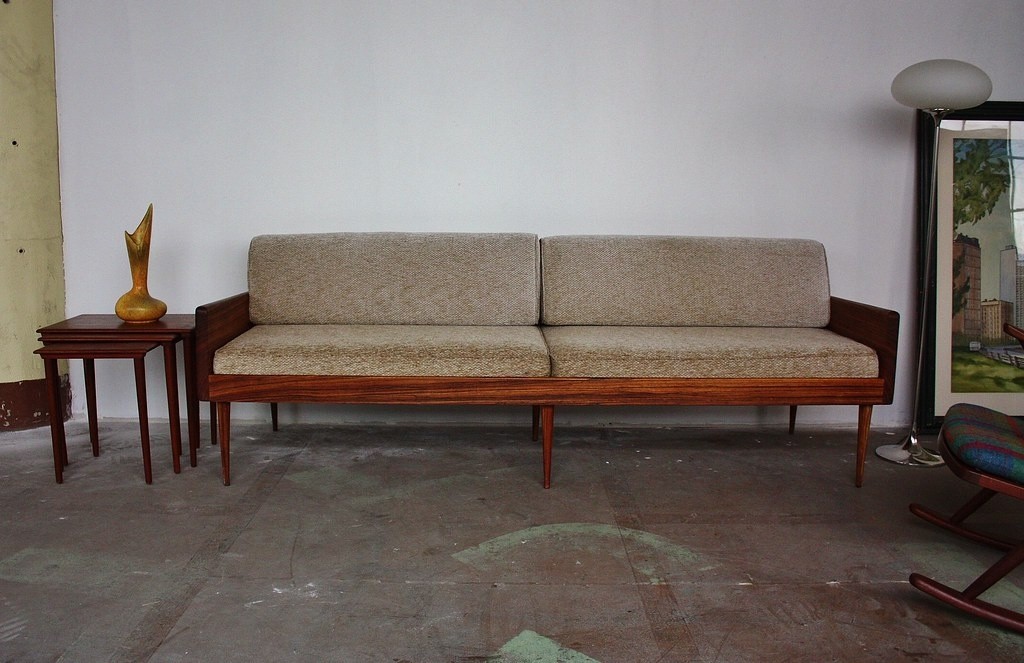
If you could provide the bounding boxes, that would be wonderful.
[914,100,1024,435]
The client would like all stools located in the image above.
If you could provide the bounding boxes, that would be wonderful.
[38,335,201,472]
[34,345,183,485]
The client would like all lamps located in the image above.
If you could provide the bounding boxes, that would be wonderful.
[876,58,992,469]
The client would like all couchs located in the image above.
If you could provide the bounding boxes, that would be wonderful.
[195,234,900,487]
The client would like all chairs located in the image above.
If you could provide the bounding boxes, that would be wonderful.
[908,402,1024,635]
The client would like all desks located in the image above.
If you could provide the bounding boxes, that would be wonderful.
[36,314,217,467]
